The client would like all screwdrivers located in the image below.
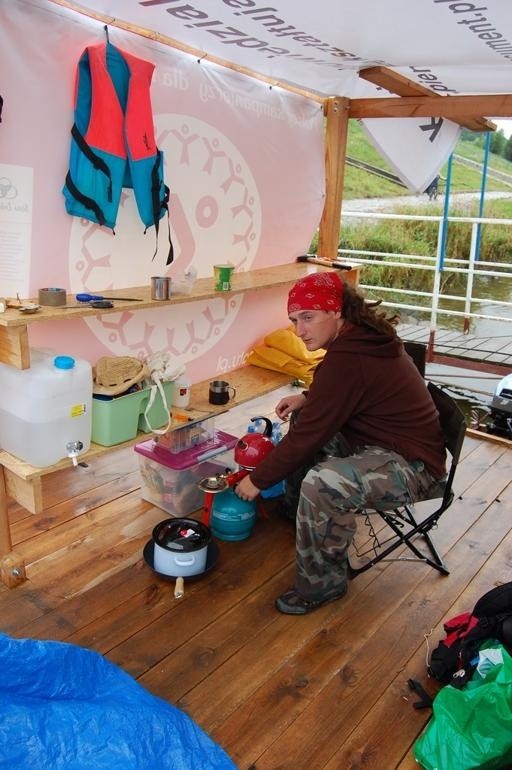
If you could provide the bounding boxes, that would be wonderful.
[76,294,145,302]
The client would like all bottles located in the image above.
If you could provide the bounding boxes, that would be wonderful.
[246,419,285,495]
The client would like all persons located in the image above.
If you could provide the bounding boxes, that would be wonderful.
[235,271,450,617]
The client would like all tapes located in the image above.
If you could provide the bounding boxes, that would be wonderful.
[39,288,66,306]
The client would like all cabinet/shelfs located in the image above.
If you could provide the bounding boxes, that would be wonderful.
[0,255,371,515]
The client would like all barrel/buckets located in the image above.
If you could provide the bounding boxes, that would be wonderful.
[0,351,93,469]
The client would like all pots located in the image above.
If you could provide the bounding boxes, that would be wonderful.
[143,518,220,600]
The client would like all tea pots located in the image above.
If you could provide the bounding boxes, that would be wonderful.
[222,414,279,491]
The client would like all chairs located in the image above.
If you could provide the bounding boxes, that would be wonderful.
[341,384,476,577]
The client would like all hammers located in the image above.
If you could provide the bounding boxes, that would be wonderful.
[296,255,354,270]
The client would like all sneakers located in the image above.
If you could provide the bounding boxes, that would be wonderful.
[274,580,347,616]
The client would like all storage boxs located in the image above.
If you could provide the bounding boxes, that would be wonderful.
[131,432,238,519]
[90,379,174,448]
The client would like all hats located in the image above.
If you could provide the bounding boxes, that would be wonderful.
[91,355,150,398]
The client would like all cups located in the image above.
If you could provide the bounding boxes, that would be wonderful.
[212,264,235,291]
[150,276,170,301]
[173,383,190,409]
[209,380,237,405]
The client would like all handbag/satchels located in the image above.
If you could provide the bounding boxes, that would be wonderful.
[134,351,186,437]
[413,581,511,770]
